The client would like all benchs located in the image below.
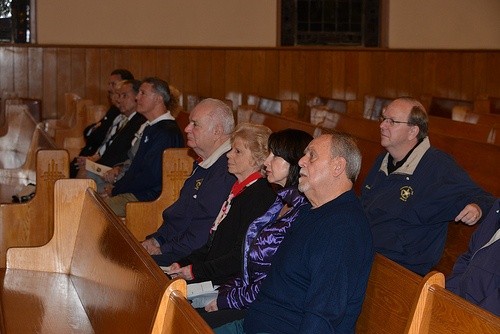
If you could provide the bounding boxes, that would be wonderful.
[0,85,500,334]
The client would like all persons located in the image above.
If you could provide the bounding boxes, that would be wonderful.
[360,98,497,279]
[69,67,315,330]
[212,133,377,334]
[445,197,500,319]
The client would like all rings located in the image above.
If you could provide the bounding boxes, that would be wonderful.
[473,215,477,219]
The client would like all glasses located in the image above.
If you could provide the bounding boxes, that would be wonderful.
[378,114,417,128]
